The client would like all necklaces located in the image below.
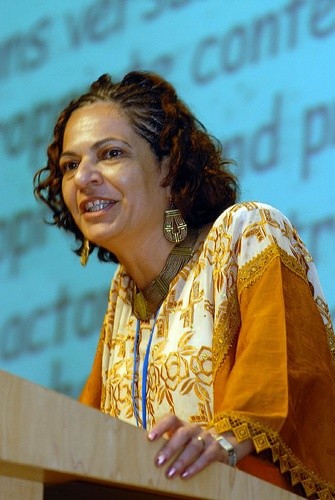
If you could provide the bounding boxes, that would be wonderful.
[131,309,160,431]
[131,226,200,323]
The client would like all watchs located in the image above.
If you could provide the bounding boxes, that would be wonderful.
[211,434,236,467]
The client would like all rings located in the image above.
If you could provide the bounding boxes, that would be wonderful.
[195,436,206,449]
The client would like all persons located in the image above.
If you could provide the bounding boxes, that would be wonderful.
[34,71,335,500]
